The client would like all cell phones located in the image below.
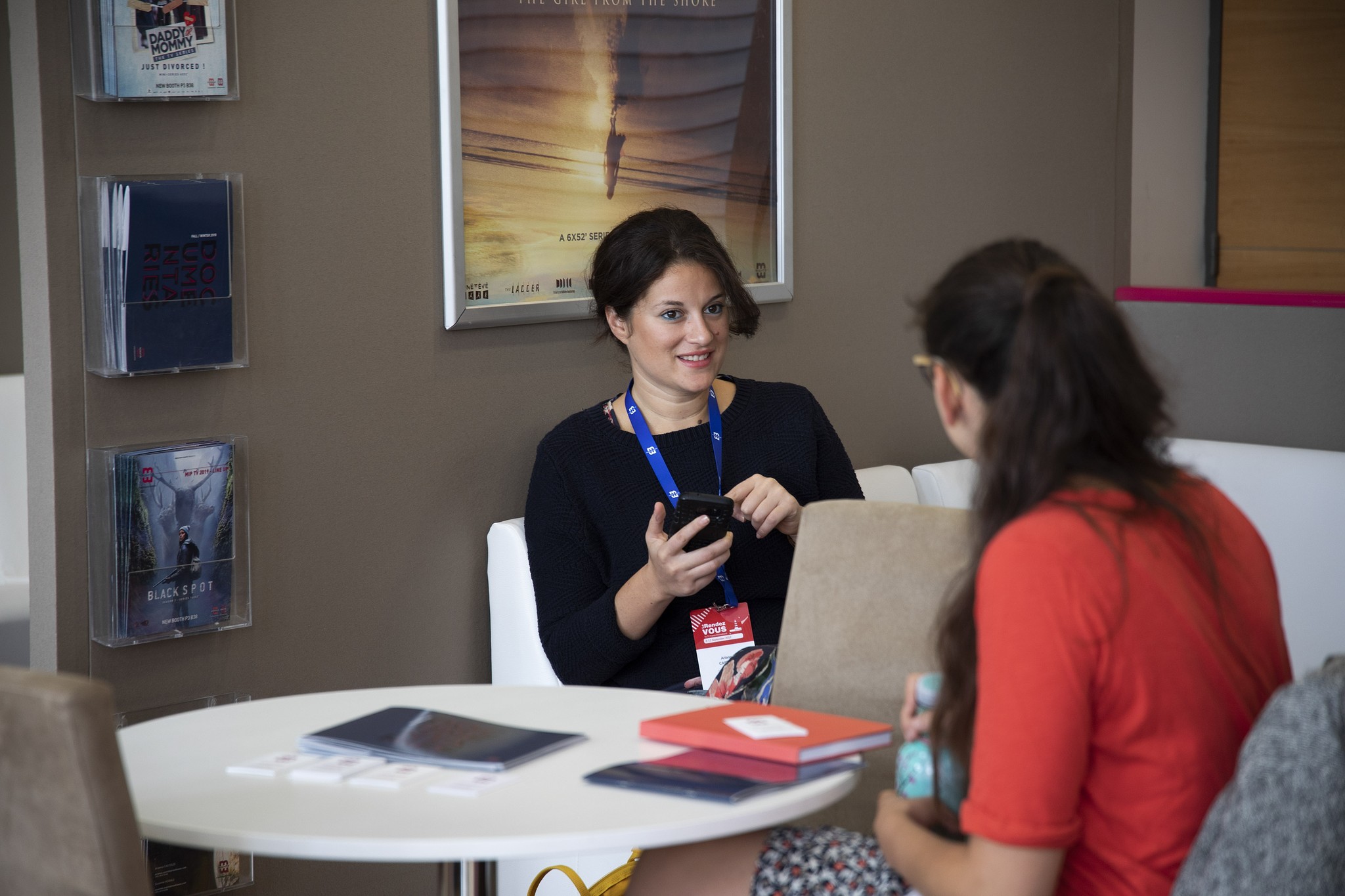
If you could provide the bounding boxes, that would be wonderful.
[667,491,736,553]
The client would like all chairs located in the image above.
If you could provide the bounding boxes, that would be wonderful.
[0,667,153,896]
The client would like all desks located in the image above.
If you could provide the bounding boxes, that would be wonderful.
[117,686,861,896]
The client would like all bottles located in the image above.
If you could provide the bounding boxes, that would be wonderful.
[894,672,966,838]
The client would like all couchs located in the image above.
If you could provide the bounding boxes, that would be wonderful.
[487,435,1344,896]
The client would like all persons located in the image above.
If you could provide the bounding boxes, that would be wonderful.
[522,207,867,706]
[624,235,1294,896]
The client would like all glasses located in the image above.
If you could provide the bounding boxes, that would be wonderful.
[912,353,962,398]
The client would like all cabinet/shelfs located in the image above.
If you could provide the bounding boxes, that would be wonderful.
[69,2,252,896]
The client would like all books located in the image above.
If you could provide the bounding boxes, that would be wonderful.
[641,700,894,768]
[90,0,236,651]
[299,707,588,772]
[583,748,863,803]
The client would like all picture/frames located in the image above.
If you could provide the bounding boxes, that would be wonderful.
[437,0,793,330]
